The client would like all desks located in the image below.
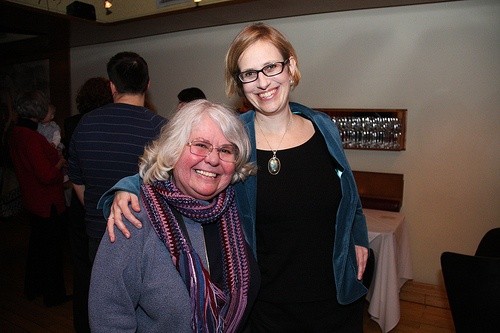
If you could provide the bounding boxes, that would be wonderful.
[362,208,412,333]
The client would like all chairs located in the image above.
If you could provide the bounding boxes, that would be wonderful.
[440,227,500,333]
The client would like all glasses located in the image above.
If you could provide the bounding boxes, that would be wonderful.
[186,140,239,162]
[238,57,289,84]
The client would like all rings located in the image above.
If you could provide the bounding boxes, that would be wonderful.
[108,217,114,219]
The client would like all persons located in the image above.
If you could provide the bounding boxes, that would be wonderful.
[99,22,370,333]
[176,87,206,111]
[62,76,114,333]
[8,86,73,309]
[67,52,167,263]
[474,227,500,258]
[85,98,258,333]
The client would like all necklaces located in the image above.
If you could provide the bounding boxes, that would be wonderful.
[256,118,292,175]
